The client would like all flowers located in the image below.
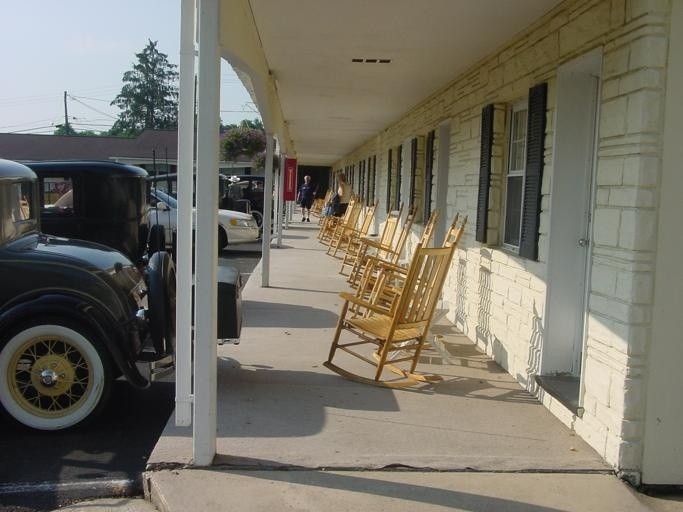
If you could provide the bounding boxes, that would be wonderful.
[219,125,279,173]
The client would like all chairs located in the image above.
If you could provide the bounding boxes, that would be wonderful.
[309,188,467,389]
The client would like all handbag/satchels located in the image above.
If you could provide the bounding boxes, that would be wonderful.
[322,206,333,217]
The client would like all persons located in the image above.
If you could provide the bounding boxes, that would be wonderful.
[296,175,315,222]
[335,171,353,217]
[254,181,261,190]
[227,176,243,200]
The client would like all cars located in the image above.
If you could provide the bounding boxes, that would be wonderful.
[46,186,259,253]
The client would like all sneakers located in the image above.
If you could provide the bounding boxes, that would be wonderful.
[302,218,310,222]
